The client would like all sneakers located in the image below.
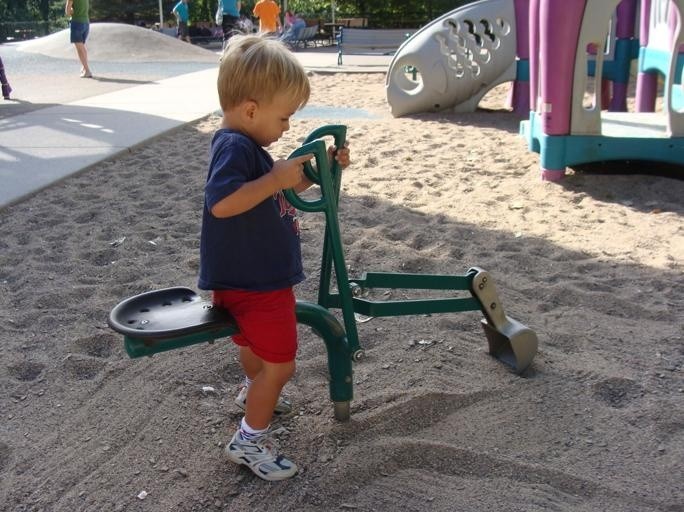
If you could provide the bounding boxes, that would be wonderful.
[224,432,298,481]
[234,387,292,413]
[79,69,92,78]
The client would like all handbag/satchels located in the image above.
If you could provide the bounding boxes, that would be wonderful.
[216,8,223,24]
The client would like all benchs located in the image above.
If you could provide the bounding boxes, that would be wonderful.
[287,24,318,52]
[336,25,423,66]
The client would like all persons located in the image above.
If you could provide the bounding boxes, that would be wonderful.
[0,57,13,101]
[172,0,191,42]
[216,0,243,48]
[283,10,295,33]
[198,22,351,482]
[280,14,305,45]
[64,1,94,79]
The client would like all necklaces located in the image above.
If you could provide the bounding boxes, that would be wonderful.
[253,0,284,38]
